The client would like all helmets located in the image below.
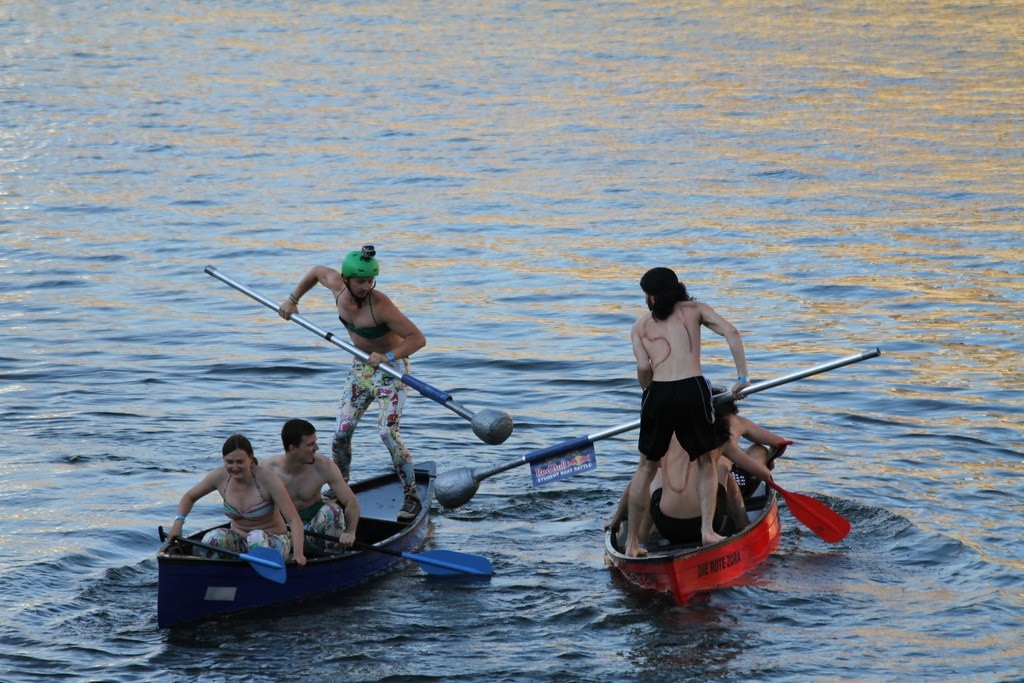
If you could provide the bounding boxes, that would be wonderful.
[341,251,379,278]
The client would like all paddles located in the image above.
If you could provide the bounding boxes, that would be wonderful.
[159,525,286,584]
[733,464,852,543]
[741,440,794,496]
[286,526,491,576]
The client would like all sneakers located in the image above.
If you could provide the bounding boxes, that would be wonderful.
[397,497,422,519]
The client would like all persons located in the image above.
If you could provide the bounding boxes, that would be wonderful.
[166,434,307,565]
[280,246,426,520]
[604,384,788,544]
[257,418,360,556]
[623,268,749,558]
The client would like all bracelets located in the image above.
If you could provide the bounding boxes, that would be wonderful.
[175,514,185,520]
[391,350,398,360]
[737,376,750,382]
[385,352,395,362]
[289,294,298,304]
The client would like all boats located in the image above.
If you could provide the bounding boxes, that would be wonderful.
[156,461,437,629]
[605,445,781,603]
